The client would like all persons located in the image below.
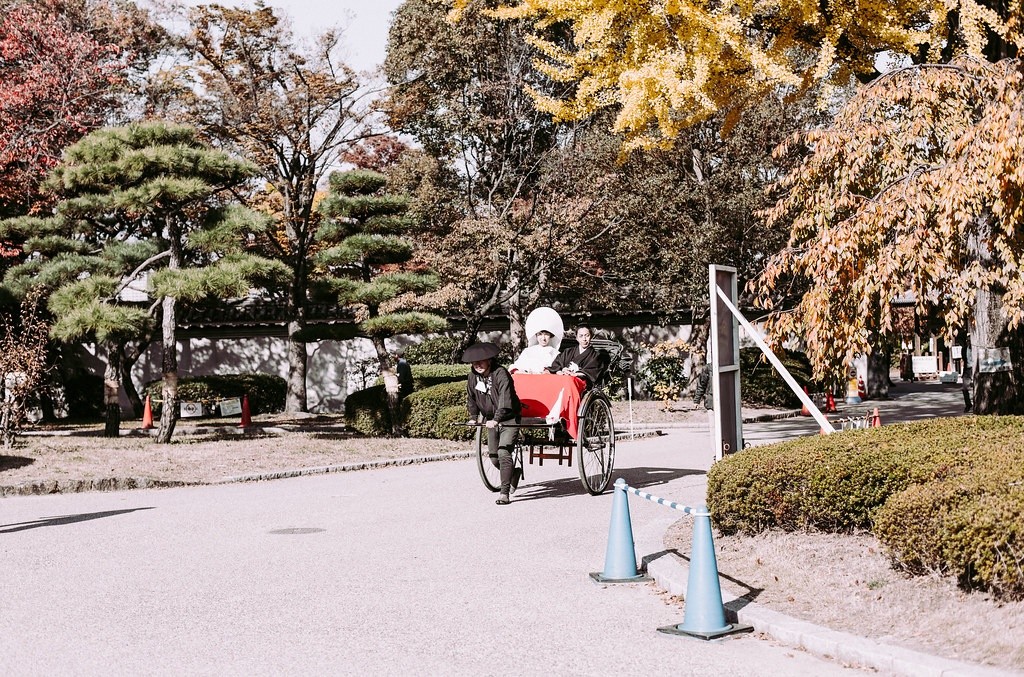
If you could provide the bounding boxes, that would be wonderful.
[538,323,605,394]
[460,342,523,504]
[506,322,563,375]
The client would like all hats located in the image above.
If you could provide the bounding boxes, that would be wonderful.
[525,306,565,351]
[461,342,501,362]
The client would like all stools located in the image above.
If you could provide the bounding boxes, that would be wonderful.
[530,438,573,468]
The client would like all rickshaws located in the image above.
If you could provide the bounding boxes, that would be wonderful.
[455,337,625,495]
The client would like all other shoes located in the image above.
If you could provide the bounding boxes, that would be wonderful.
[495,493,509,505]
[509,468,522,494]
[963,404,972,413]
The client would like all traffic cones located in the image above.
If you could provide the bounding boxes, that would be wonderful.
[857,375,870,400]
[872,408,881,427]
[654,506,756,642]
[136,396,158,430]
[820,415,828,434]
[236,395,255,429]
[799,387,813,417]
[821,389,843,414]
[588,476,656,586]
